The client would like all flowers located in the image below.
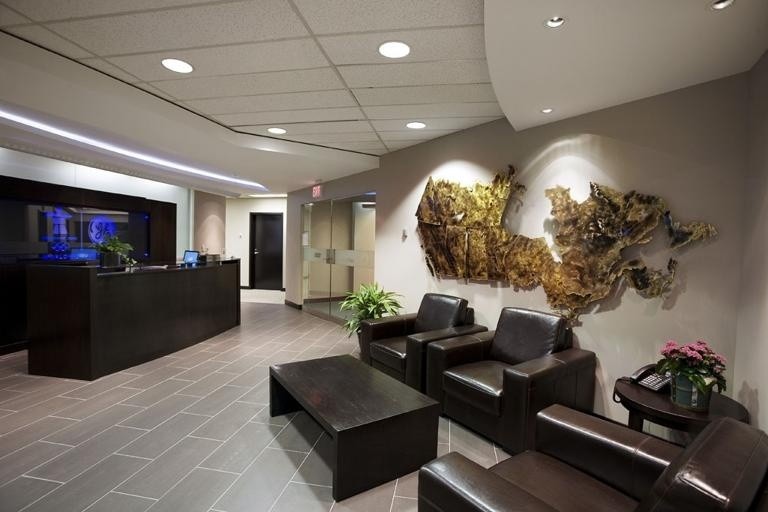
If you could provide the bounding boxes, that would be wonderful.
[656,338,727,396]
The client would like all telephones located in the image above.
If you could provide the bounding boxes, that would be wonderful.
[630,364,672,392]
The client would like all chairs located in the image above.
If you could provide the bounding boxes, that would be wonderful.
[360,293,488,391]
[418,404,768,512]
[427,307,596,454]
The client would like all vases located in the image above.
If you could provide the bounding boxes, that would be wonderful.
[669,374,712,410]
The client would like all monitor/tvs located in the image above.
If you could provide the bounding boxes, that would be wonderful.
[183,249,200,262]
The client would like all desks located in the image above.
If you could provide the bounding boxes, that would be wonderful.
[615,375,749,433]
